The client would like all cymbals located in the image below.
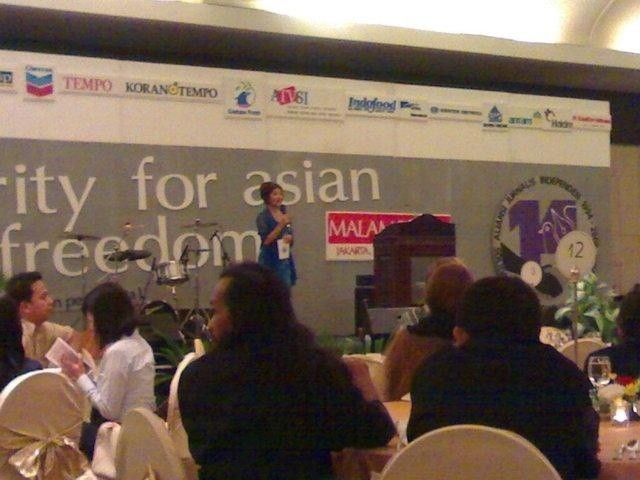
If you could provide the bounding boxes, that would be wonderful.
[103,250,151,262]
[112,224,145,231]
[179,222,218,227]
[59,230,101,240]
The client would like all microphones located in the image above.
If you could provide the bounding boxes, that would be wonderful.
[280,204,292,234]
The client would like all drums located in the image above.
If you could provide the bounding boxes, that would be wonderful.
[140,298,180,341]
[154,261,189,286]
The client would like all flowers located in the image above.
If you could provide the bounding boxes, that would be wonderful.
[599,374,640,404]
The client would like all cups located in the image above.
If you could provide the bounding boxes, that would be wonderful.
[588,356,611,389]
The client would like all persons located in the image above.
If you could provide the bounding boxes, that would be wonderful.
[63,282,153,431]
[256,181,297,287]
[584,283,639,379]
[374,263,474,401]
[405,275,599,480]
[2,271,76,389]
[178,261,396,478]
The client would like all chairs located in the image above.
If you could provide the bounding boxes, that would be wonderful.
[353,355,391,404]
[1,368,97,480]
[379,424,561,478]
[559,338,604,373]
[159,352,201,479]
[115,407,189,479]
